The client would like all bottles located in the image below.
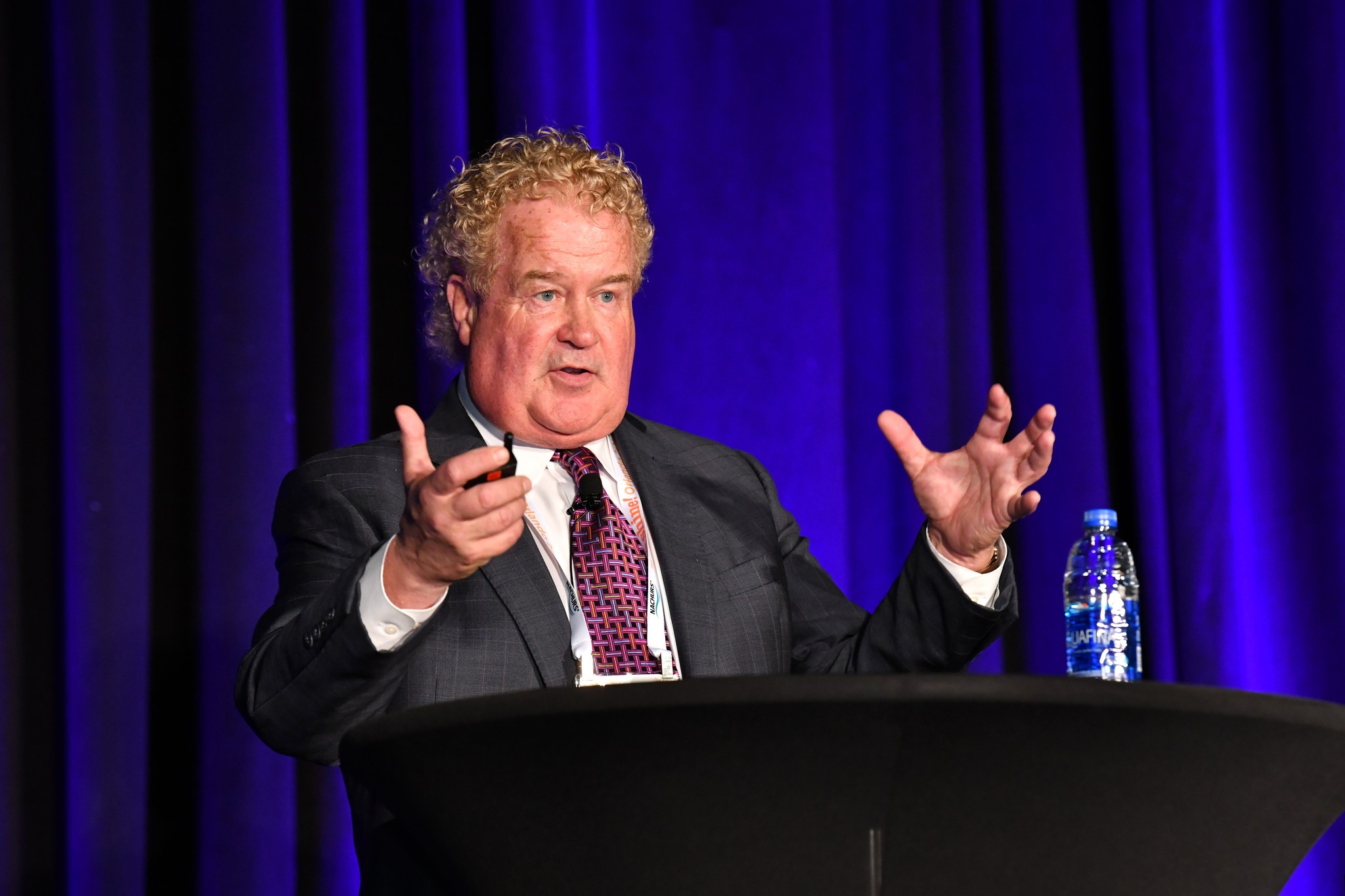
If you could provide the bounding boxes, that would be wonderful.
[1064,509,1143,683]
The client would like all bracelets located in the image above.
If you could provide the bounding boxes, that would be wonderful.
[977,541,1004,575]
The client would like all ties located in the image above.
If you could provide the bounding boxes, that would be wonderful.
[547,442,678,676]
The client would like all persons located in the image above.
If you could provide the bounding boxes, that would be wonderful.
[233,113,1059,769]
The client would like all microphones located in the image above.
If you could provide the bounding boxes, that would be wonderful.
[579,472,602,510]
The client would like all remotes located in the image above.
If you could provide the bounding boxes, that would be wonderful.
[464,431,518,489]
